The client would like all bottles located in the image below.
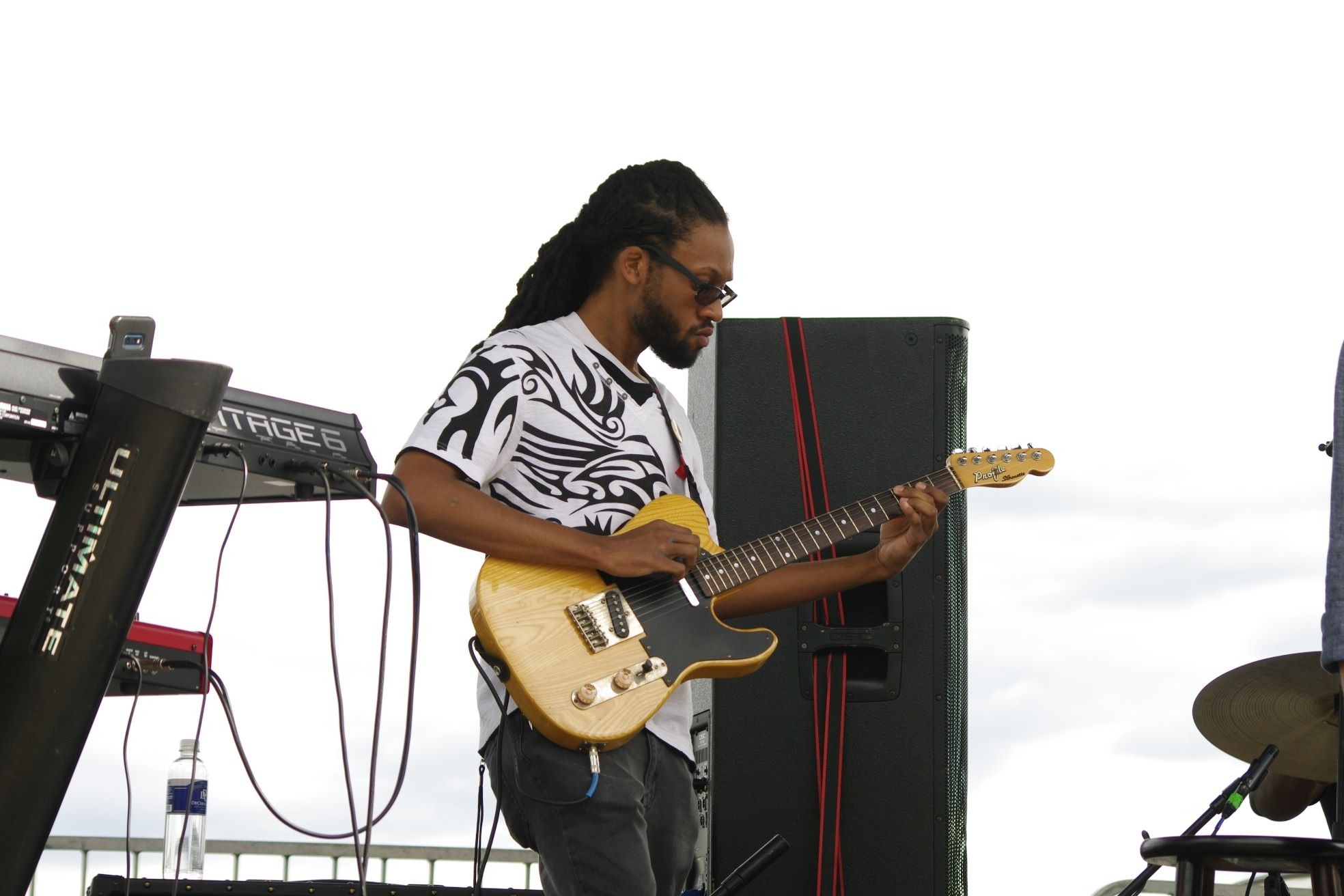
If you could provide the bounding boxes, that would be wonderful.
[162,739,209,881]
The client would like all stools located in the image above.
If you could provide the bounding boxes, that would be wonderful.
[1141,835,1344,896]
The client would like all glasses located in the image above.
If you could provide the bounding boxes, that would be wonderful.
[632,241,738,309]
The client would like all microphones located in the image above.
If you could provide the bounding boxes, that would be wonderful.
[1222,743,1281,818]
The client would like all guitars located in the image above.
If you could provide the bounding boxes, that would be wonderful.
[468,445,1054,749]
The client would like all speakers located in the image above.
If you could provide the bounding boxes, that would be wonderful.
[686,316,967,895]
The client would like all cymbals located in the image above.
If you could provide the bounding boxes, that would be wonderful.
[1192,651,1339,783]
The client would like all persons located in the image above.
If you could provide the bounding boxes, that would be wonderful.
[380,158,952,896]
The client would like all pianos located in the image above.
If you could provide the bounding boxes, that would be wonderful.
[0,333,378,896]
